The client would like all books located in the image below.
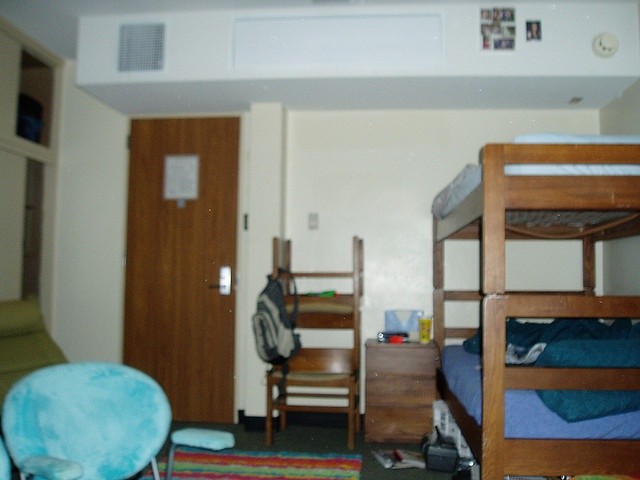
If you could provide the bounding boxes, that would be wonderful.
[394,448,427,470]
[373,447,396,469]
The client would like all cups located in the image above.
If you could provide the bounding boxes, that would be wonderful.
[418,314,433,345]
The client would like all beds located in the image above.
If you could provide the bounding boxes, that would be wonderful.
[431,135,639,480]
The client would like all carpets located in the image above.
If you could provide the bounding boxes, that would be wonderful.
[134,442,362,479]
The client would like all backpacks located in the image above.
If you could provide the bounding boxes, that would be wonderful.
[252,269,301,363]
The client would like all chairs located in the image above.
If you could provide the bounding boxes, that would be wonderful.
[0,362,175,478]
[265,313,361,452]
[266,236,363,376]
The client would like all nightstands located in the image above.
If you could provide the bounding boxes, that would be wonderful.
[366,339,435,444]
